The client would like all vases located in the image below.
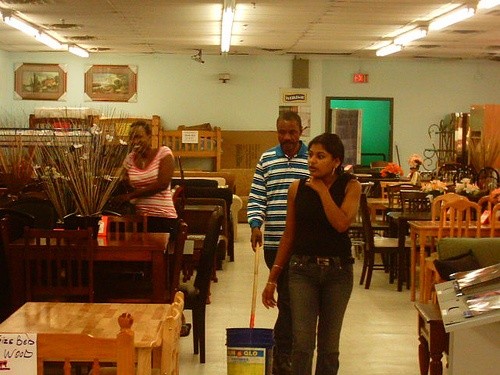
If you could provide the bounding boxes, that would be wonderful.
[63,214,102,239]
[411,169,419,178]
[431,191,444,204]
[387,172,400,178]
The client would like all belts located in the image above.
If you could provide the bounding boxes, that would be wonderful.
[297,256,354,267]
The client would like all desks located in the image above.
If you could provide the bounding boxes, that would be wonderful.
[365,197,408,222]
[7,232,170,305]
[407,221,500,302]
[383,210,467,291]
[359,177,411,181]
[0,301,170,375]
[415,304,450,375]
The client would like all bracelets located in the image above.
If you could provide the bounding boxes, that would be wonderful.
[272,264,284,271]
[266,282,277,287]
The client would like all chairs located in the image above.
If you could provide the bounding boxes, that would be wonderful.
[0,208,226,375]
[350,164,499,303]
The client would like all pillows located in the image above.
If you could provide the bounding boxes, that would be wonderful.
[433,250,482,280]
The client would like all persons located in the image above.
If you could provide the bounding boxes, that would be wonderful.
[262,132,362,375]
[246,110,311,375]
[102,121,179,241]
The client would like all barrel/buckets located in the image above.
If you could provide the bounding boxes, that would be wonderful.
[225,328,274,375]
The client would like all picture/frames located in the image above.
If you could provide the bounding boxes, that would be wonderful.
[13,63,67,101]
[83,64,138,102]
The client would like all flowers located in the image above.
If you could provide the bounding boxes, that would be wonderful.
[455,177,480,195]
[408,155,422,168]
[422,180,448,193]
[33,107,142,212]
[380,163,404,177]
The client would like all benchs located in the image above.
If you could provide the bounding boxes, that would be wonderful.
[183,198,228,270]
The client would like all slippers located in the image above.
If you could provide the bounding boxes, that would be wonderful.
[180,324,191,336]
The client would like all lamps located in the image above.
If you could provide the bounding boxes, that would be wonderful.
[376,44,402,58]
[429,3,476,31]
[68,43,90,59]
[394,26,428,46]
[9,14,62,52]
[221,0,236,53]
[478,0,500,9]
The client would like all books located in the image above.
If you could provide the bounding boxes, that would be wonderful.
[449,263,500,317]
